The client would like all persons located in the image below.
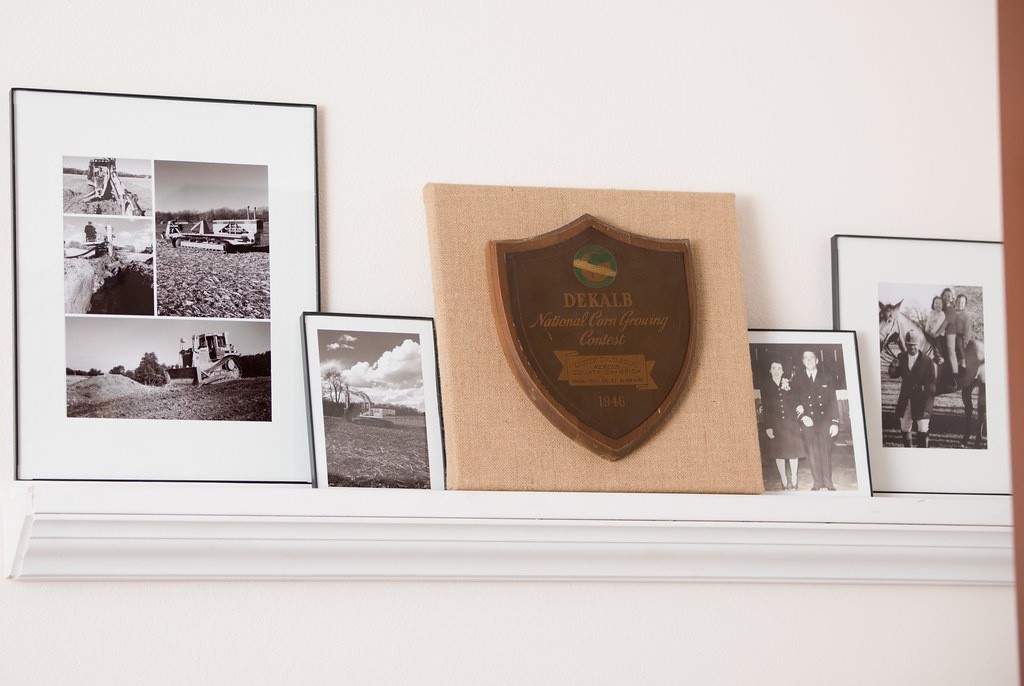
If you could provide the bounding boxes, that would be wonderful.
[760,348,840,493]
[888,287,971,449]
[84,221,98,242]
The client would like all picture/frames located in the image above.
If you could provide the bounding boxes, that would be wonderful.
[748,328,874,496]
[832,233,1013,494]
[300,312,449,489]
[10,87,321,485]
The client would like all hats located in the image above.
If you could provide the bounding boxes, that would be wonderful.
[906,330,919,344]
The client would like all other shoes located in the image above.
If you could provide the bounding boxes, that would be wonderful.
[782,481,788,491]
[827,487,836,492]
[934,356,945,364]
[791,481,798,491]
[810,485,825,491]
[959,361,967,370]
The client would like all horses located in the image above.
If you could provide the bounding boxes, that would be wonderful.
[878,297,985,449]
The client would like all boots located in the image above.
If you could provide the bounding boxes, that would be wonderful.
[950,372,960,390]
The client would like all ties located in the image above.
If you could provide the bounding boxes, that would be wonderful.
[809,373,813,382]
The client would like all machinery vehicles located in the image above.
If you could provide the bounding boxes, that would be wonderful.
[80,157,146,216]
[162,205,264,253]
[341,383,396,429]
[164,331,243,385]
